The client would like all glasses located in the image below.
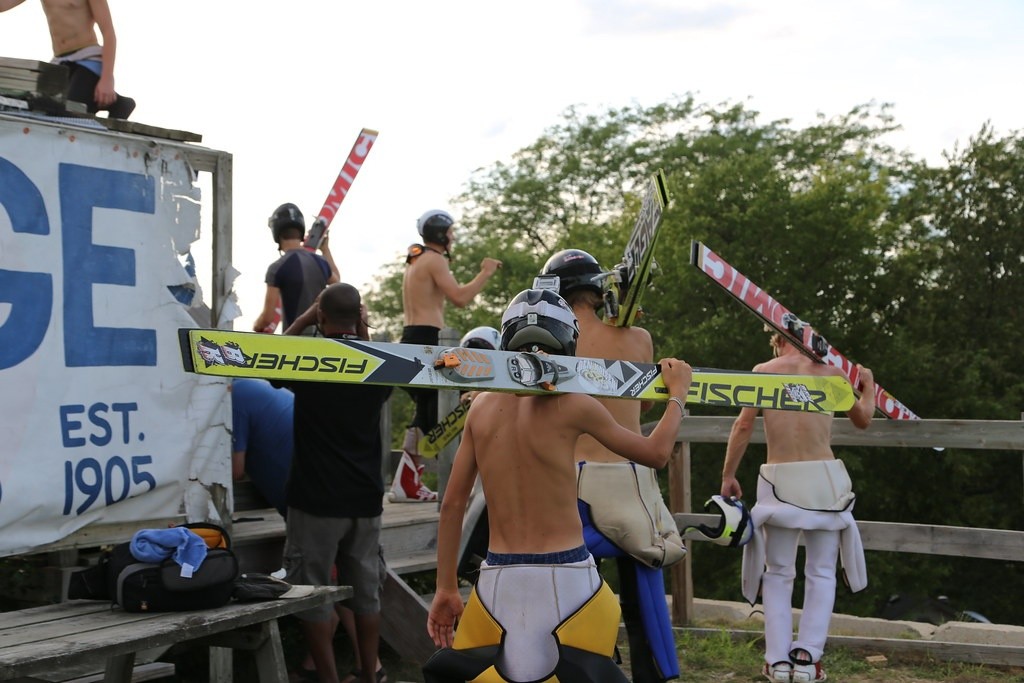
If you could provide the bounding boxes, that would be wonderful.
[406,243,423,263]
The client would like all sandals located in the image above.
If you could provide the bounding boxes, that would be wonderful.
[341,665,387,683]
[290,666,319,683]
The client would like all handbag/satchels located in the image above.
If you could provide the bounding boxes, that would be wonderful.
[101,521,240,614]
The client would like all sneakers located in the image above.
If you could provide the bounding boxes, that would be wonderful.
[761,662,791,682]
[792,663,827,682]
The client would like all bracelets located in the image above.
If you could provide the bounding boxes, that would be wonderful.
[668,396,686,421]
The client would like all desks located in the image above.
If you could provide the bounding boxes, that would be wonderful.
[0,585,354,683]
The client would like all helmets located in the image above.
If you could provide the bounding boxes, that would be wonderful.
[679,495,753,546]
[266,202,303,240]
[500,288,580,355]
[415,208,453,244]
[459,326,501,351]
[541,248,603,292]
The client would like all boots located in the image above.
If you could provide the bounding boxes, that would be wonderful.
[388,451,439,503]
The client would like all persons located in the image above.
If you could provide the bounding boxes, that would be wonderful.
[420,287,693,683]
[387,208,503,504]
[0,0,136,122]
[252,199,341,341]
[529,247,689,683]
[456,327,503,402]
[720,329,876,683]
[259,282,396,683]
[232,377,391,682]
